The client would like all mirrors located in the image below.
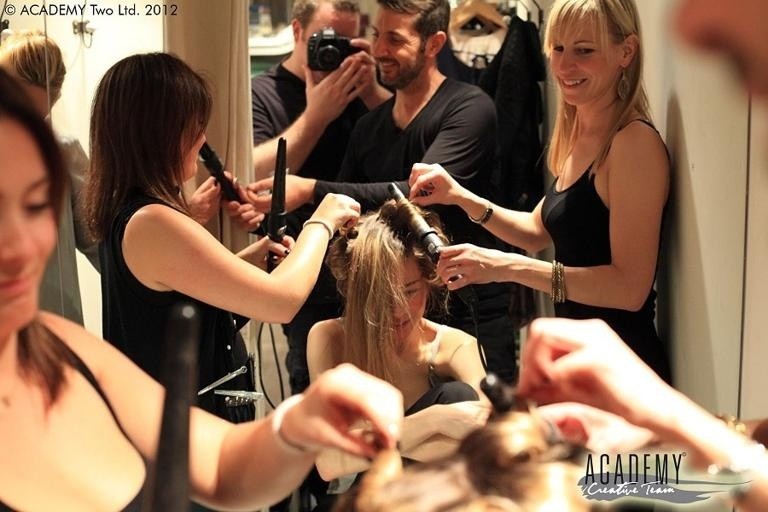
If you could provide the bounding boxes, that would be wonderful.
[1,0,181,342]
[237,2,755,425]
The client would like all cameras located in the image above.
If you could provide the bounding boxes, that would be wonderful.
[307,28,363,71]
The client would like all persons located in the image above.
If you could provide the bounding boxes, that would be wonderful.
[0,0,768,512]
